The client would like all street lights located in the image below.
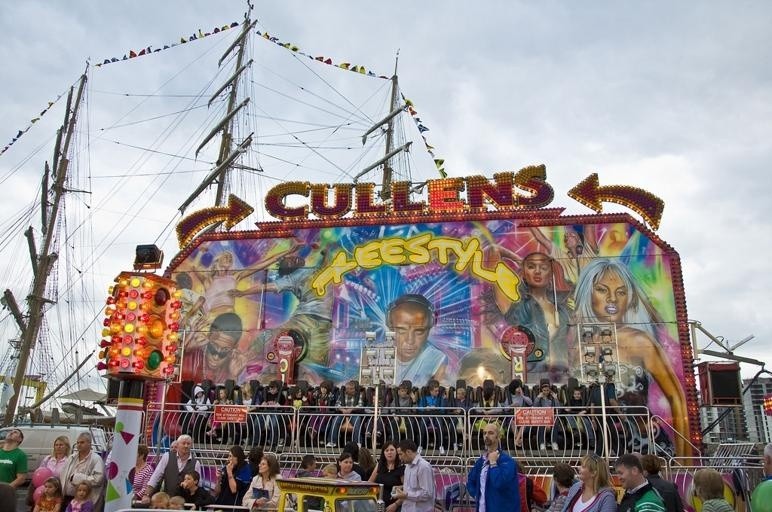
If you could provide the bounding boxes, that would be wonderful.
[155,327,187,462]
[358,330,396,461]
[575,322,621,464]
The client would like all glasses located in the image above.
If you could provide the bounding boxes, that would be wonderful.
[207,343,227,359]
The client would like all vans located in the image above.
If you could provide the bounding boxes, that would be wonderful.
[1,424,111,487]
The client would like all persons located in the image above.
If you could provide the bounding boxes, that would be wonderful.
[0,378,772,512]
